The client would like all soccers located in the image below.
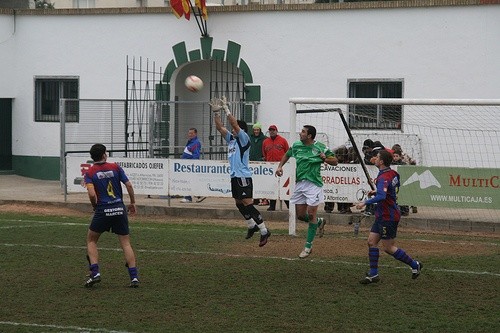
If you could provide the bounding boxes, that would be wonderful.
[185,76,203,92]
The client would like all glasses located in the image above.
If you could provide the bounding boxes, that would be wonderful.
[269,128,275,131]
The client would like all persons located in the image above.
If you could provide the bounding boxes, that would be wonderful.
[348,147,359,208]
[371,141,395,165]
[249,121,270,206]
[323,144,349,213]
[393,151,408,215]
[262,124,290,211]
[206,94,272,247]
[355,150,423,284]
[391,144,418,213]
[180,127,206,203]
[362,139,374,215]
[274,125,339,258]
[83,143,141,289]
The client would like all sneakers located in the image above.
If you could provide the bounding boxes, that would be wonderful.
[411,260,423,280]
[127,277,139,288]
[298,245,312,258]
[259,228,271,246]
[318,218,327,238]
[245,224,260,239]
[83,273,102,288]
[359,272,380,285]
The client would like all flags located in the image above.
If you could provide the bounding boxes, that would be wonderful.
[169,0,190,21]
[195,0,208,20]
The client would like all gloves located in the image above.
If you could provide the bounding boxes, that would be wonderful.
[207,96,223,117]
[217,94,231,116]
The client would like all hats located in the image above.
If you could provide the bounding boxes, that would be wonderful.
[252,121,261,129]
[269,125,277,130]
